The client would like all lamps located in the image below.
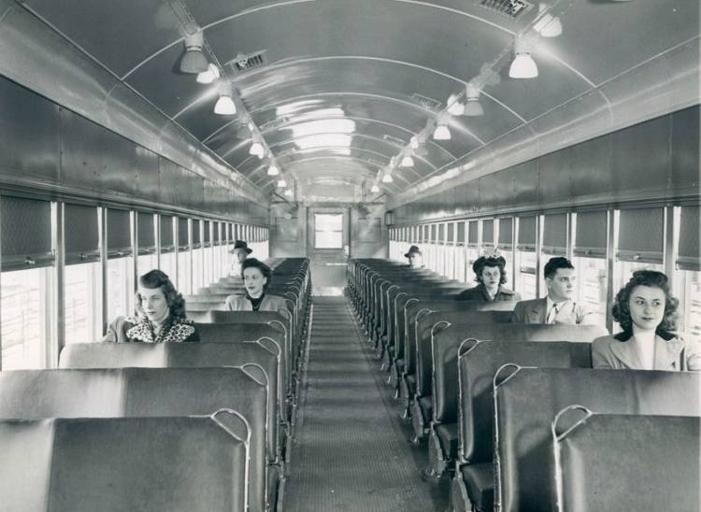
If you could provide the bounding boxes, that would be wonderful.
[370,2,539,193]
[166,1,290,189]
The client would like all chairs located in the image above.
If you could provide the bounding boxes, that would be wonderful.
[0,254,312,511]
[345,257,701,512]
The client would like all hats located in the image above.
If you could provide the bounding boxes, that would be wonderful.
[404,246,422,258]
[228,240,252,255]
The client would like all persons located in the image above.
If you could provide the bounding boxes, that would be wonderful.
[103,267,202,342]
[222,258,290,321]
[510,256,596,325]
[590,270,701,371]
[402,243,426,270]
[226,239,253,277]
[455,246,523,302]
